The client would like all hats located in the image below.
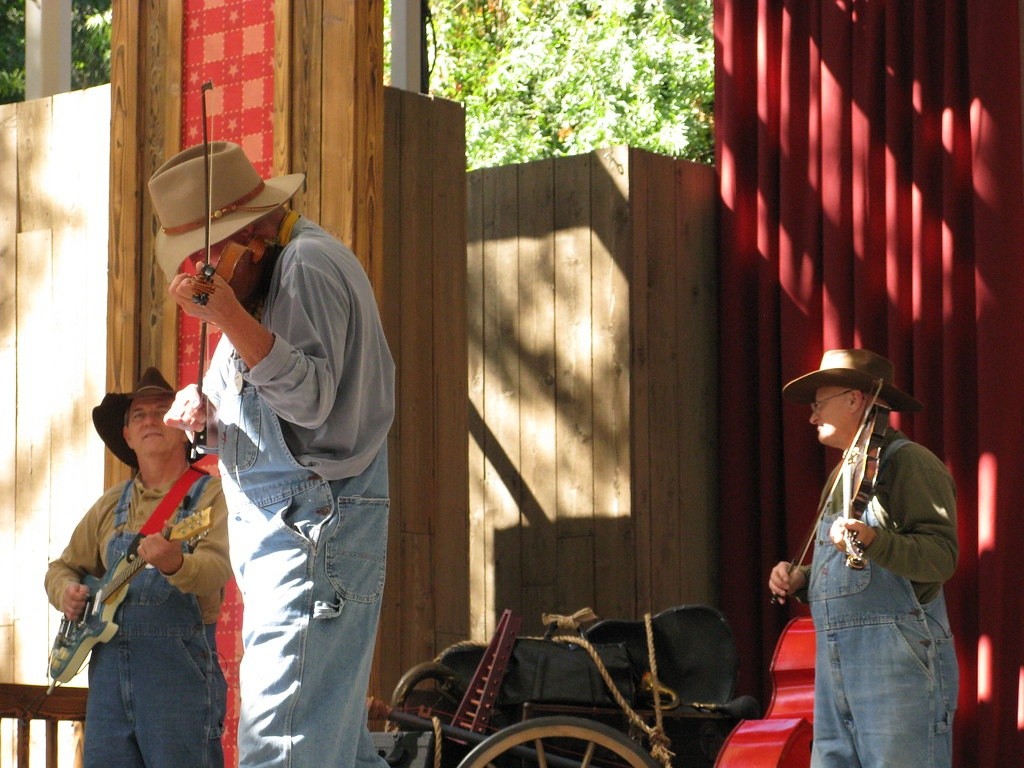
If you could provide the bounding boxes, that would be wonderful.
[92,367,208,470]
[148,140,305,284]
[781,348,924,414]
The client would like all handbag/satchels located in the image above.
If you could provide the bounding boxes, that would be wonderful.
[498,621,637,710]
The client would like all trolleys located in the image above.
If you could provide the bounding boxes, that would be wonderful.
[364,623,737,768]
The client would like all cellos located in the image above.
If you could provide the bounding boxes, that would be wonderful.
[713,614,816,768]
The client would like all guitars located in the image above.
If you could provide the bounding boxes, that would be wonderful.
[47,504,216,684]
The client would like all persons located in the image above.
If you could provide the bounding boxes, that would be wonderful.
[770,349,960,768]
[45,368,233,768]
[148,142,396,768]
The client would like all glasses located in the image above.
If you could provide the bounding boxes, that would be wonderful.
[810,387,864,412]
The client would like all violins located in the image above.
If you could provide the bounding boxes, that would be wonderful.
[190,232,274,307]
[841,401,893,570]
